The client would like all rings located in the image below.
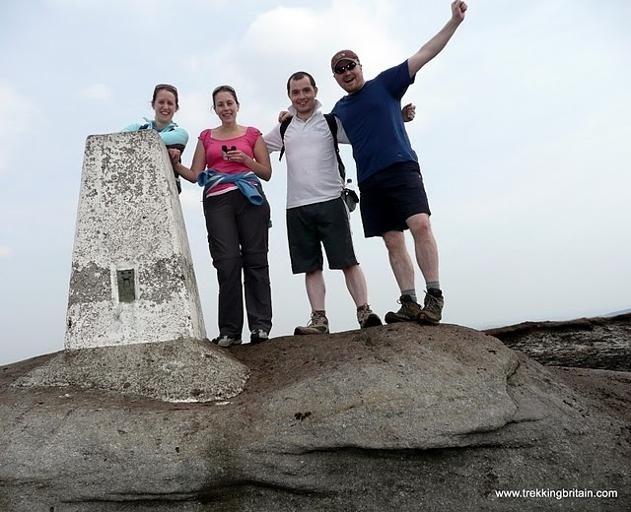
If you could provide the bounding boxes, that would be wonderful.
[239,152,241,157]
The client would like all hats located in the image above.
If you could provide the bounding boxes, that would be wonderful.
[331,50,359,72]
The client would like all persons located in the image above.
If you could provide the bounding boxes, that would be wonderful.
[122,83,189,196]
[167,85,274,347]
[261,71,417,336]
[277,0,469,325]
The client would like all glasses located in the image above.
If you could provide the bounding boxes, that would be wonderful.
[334,62,359,74]
[156,85,177,92]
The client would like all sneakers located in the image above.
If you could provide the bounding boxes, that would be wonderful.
[415,290,444,325]
[212,334,241,348]
[295,311,329,335]
[385,295,422,324]
[250,328,268,343]
[357,303,382,329]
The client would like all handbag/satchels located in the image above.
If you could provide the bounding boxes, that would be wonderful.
[340,187,359,213]
